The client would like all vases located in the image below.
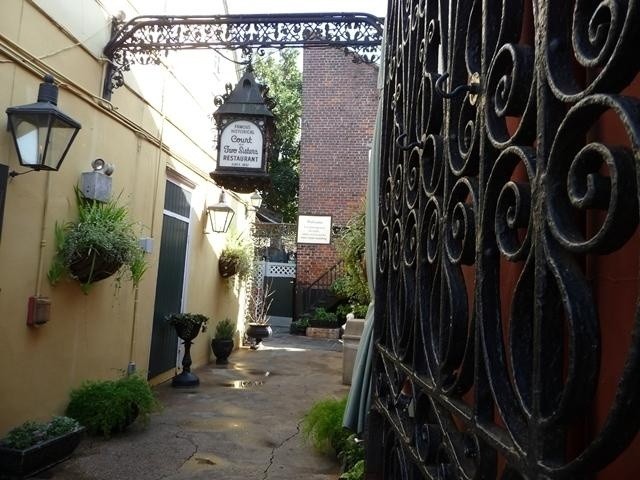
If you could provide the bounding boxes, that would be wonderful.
[246,321,273,349]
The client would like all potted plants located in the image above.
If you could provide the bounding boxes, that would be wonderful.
[290,318,309,336]
[67,373,163,438]
[218,245,252,295]
[162,312,209,389]
[3,415,90,473]
[44,188,149,292]
[301,394,351,456]
[340,206,370,386]
[337,305,353,326]
[309,307,339,328]
[211,318,236,366]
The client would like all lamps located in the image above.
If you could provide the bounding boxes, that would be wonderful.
[80,156,116,205]
[4,74,82,173]
[205,187,236,234]
[210,51,280,193]
[245,189,263,213]
